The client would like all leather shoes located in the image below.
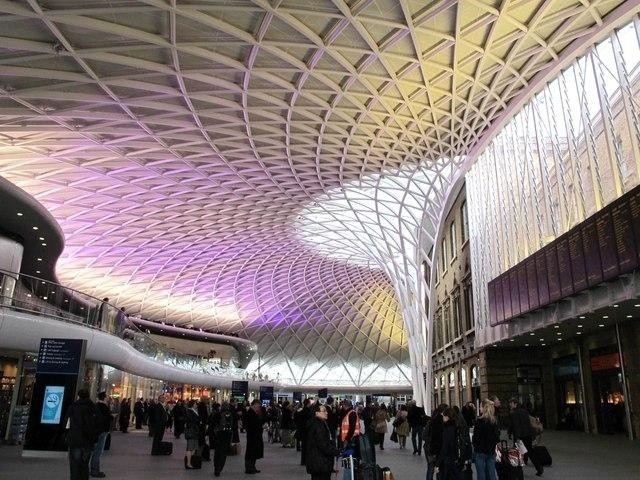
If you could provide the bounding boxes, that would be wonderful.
[246,471,256,474]
[254,469,261,473]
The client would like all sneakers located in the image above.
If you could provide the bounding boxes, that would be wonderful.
[91,471,103,475]
[93,473,104,477]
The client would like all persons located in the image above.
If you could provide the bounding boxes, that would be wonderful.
[213,402,233,476]
[140,397,145,425]
[64,388,104,480]
[488,394,503,442]
[197,396,212,461]
[267,395,412,417]
[299,399,310,466]
[127,397,132,427]
[303,403,341,480]
[207,409,218,448]
[461,402,474,428]
[245,399,265,475]
[339,398,360,463]
[224,409,231,430]
[183,399,203,469]
[420,403,448,480]
[375,403,391,450]
[507,399,520,447]
[89,390,118,478]
[434,409,458,480]
[393,404,409,449]
[133,397,144,429]
[144,396,250,412]
[515,404,550,477]
[453,405,474,479]
[229,398,240,444]
[120,398,129,434]
[472,398,501,480]
[149,394,169,455]
[407,400,429,455]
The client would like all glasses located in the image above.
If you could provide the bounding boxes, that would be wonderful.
[319,409,328,413]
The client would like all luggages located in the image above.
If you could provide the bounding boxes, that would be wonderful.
[375,466,383,480]
[358,464,375,479]
[280,428,294,448]
[356,435,376,465]
[341,456,354,480]
[532,445,553,466]
[496,441,524,480]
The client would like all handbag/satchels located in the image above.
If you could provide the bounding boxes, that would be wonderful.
[392,410,404,427]
[226,444,241,455]
[383,468,394,480]
[529,414,545,439]
[419,407,429,426]
[390,426,399,443]
[159,441,173,455]
[184,456,201,468]
